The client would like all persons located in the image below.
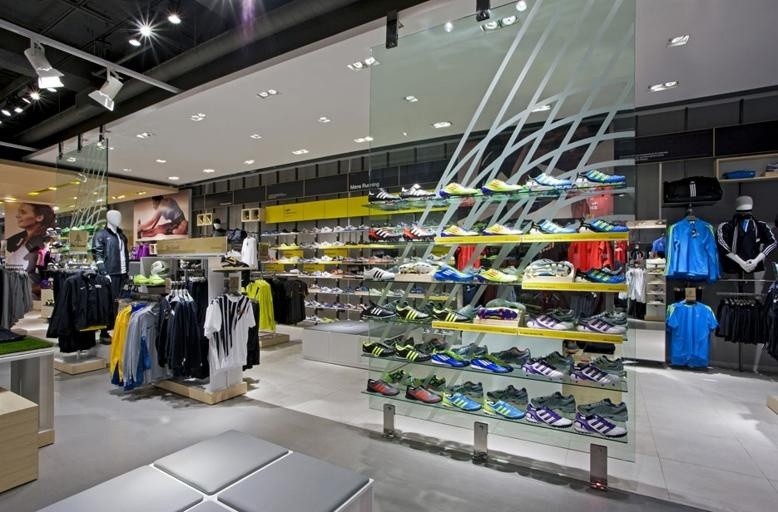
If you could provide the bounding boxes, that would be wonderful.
[136,195,188,238]
[716,195,776,275]
[91,210,130,344]
[7,202,55,266]
[211,219,225,237]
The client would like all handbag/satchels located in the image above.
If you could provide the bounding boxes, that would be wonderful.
[664,176,723,202]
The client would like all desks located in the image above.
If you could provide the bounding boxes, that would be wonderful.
[0,335,56,449]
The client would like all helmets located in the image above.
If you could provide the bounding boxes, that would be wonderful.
[150,259,170,276]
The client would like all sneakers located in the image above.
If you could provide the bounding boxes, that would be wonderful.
[149,274,165,285]
[219,258,248,269]
[367,254,625,283]
[133,274,149,284]
[362,333,630,437]
[370,215,629,242]
[258,224,369,323]
[360,300,629,334]
[367,168,628,203]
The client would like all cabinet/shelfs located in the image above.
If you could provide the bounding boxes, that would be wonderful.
[259,229,457,340]
[360,186,636,492]
[643,262,666,321]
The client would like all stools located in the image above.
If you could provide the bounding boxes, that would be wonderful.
[0,385,41,495]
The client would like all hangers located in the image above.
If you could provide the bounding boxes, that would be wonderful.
[165,280,195,303]
[224,281,241,296]
[720,292,757,307]
[687,208,698,221]
[681,297,698,305]
[83,270,98,277]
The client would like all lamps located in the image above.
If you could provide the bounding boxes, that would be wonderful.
[22,31,126,112]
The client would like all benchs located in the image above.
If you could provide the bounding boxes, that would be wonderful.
[33,428,376,512]
